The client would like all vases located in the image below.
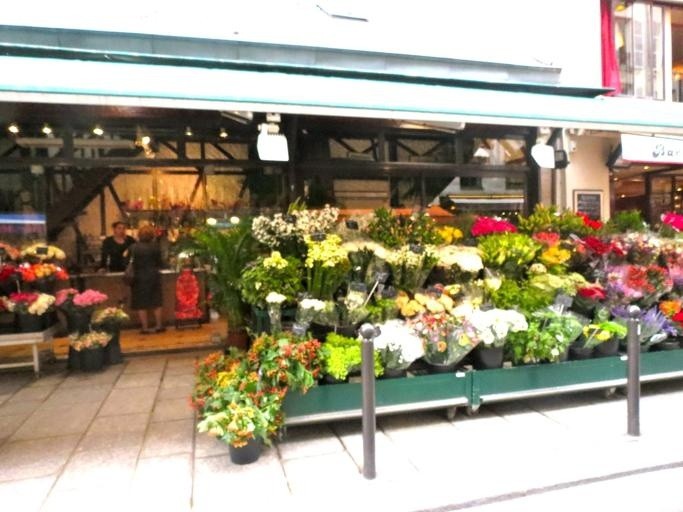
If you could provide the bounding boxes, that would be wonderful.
[19,264,70,283]
[394,288,453,318]
[408,314,480,366]
[237,251,300,311]
[434,245,483,282]
[623,231,662,266]
[659,209,683,238]
[21,243,66,261]
[73,290,109,331]
[90,306,129,332]
[359,318,425,371]
[265,292,286,336]
[670,308,683,330]
[385,242,440,290]
[303,233,352,300]
[72,332,112,351]
[573,282,608,314]
[451,304,529,348]
[187,348,240,413]
[576,235,623,268]
[576,210,604,230]
[583,321,627,349]
[240,334,322,409]
[667,257,683,286]
[611,304,677,346]
[451,277,502,311]
[1,292,55,315]
[292,298,326,336]
[292,204,342,257]
[469,215,517,237]
[0,242,19,261]
[539,246,572,273]
[510,314,555,365]
[657,299,681,317]
[530,230,560,248]
[527,263,585,291]
[608,263,673,305]
[196,396,281,448]
[54,288,78,314]
[476,231,535,273]
[659,237,683,272]
[432,223,464,247]
[532,305,575,359]
[250,213,295,251]
[0,263,15,285]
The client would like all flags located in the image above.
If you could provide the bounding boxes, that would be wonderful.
[228,332,249,351]
[80,350,104,372]
[64,312,89,334]
[0,281,20,296]
[659,341,680,351]
[381,367,403,378]
[230,439,262,465]
[428,362,455,371]
[639,344,652,353]
[326,375,347,383]
[31,280,56,294]
[310,321,353,344]
[571,342,596,357]
[599,339,620,356]
[480,348,504,369]
[69,344,81,370]
[560,345,571,362]
[14,310,49,332]
[103,332,122,364]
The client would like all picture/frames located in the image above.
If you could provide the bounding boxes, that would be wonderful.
[69,266,208,331]
[0,322,62,377]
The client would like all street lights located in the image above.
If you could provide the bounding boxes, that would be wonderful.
[123,244,136,287]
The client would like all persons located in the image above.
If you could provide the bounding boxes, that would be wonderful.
[98,221,137,273]
[122,227,162,334]
